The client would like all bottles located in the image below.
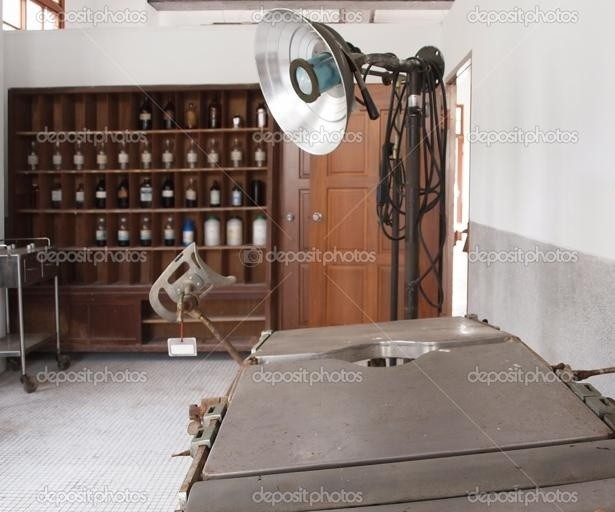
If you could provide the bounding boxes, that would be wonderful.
[23,136,265,171]
[137,91,268,134]
[25,174,264,207]
[90,211,268,248]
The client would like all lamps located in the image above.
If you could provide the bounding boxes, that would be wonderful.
[254,7,444,366]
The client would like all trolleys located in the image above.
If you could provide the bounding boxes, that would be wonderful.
[0,233,72,395]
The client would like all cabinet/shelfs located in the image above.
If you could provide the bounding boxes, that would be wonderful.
[7,83,279,352]
